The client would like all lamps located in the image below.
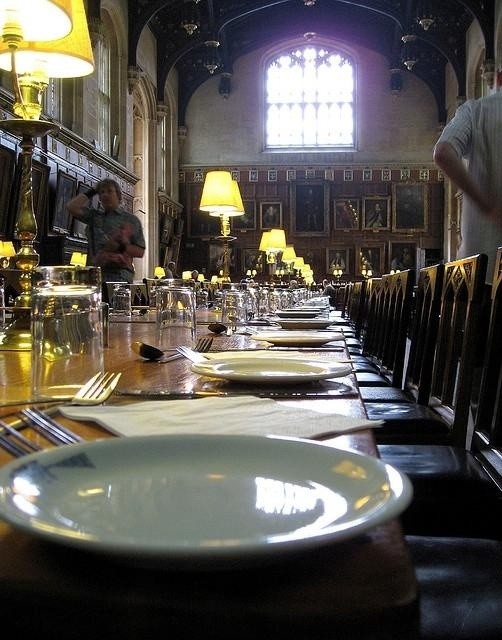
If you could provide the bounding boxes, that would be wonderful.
[182,0,233,101]
[389,0,435,94]
[302,0,318,42]
[0,0,96,349]
[198,169,316,285]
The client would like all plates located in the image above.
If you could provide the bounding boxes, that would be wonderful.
[276,302,332,327]
[1,432,414,560]
[192,356,356,387]
[248,327,345,347]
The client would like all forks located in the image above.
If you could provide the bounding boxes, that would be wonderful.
[0,369,124,436]
[13,399,78,447]
[176,346,211,365]
[0,416,37,460]
[160,338,214,363]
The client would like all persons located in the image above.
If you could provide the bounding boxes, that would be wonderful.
[188,270,204,288]
[329,252,346,273]
[66,179,145,310]
[133,286,147,314]
[392,244,416,272]
[366,202,385,226]
[431,51,502,293]
[264,207,279,228]
[162,261,176,279]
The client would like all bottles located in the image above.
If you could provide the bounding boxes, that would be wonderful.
[101,301,110,349]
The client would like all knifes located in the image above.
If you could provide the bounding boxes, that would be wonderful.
[110,387,363,404]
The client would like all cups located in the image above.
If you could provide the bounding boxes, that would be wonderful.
[221,289,250,328]
[247,289,311,318]
[31,262,110,401]
[148,283,223,306]
[156,286,197,351]
[112,287,133,318]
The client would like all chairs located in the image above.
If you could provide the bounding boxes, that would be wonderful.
[327,246,501,595]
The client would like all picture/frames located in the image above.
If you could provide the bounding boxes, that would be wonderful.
[326,182,429,284]
[178,170,295,277]
[324,167,445,182]
[289,178,330,237]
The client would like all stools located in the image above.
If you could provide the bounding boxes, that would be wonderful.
[1,246,306,357]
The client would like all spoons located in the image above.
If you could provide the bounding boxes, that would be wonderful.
[139,308,147,317]
[207,323,228,336]
[129,338,183,363]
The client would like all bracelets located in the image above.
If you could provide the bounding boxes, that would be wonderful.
[83,186,95,200]
[116,238,127,254]
